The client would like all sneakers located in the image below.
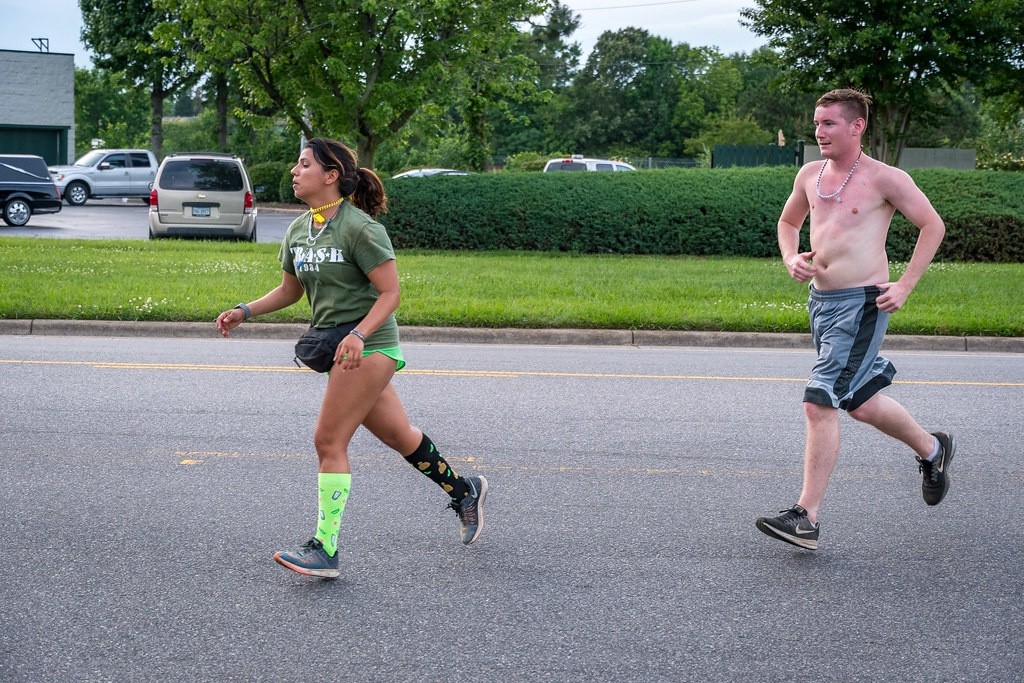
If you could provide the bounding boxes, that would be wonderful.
[915,431,958,505]
[443,475,489,544]
[273,537,340,577]
[756,504,822,551]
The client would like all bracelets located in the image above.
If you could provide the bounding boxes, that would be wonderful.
[350,330,365,341]
[234,303,251,321]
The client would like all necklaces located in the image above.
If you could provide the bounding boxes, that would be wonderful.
[816,149,861,198]
[310,198,343,223]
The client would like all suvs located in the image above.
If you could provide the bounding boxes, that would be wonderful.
[144,151,259,244]
[0,155,63,227]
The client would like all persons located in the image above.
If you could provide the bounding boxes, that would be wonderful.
[756,90,957,550]
[217,138,488,577]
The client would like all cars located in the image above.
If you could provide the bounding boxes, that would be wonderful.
[392,169,475,177]
[541,154,638,174]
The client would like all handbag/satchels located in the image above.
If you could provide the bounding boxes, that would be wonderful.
[293,315,373,373]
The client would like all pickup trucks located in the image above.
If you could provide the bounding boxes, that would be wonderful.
[51,149,160,207]
